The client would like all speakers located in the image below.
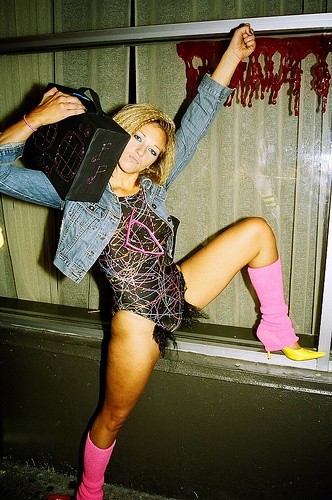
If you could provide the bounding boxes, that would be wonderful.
[26,83,129,204]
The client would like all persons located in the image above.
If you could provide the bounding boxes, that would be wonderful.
[0,20,329,500]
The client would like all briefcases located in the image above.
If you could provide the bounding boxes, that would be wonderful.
[19,82,130,202]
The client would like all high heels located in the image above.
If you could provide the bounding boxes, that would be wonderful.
[268,346,326,360]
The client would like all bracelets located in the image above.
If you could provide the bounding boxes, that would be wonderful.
[22,114,38,132]
